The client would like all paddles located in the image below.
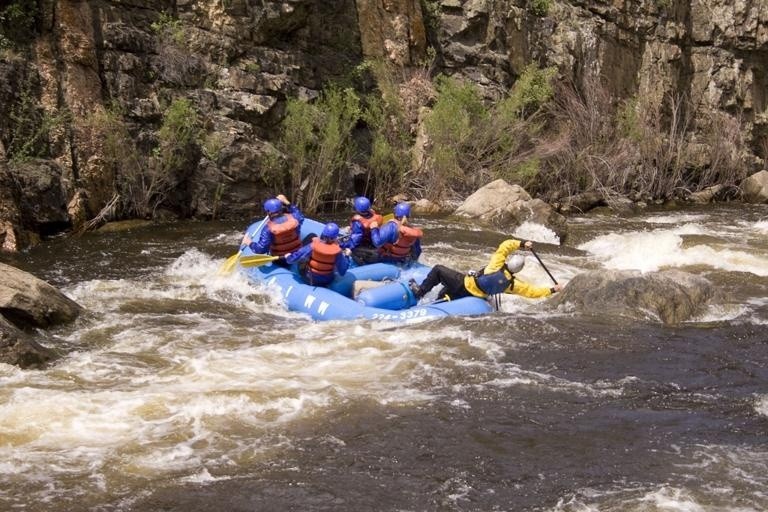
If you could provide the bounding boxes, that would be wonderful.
[336,213,394,240]
[239,254,284,269]
[218,215,269,273]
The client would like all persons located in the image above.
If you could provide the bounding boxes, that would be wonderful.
[351,203,423,267]
[239,195,316,271]
[286,222,353,287]
[338,197,383,251]
[407,238,564,304]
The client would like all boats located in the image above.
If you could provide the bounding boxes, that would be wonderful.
[236,218,496,323]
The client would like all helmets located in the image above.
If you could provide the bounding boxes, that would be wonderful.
[264,198,283,214]
[354,196,370,212]
[394,202,410,217]
[323,222,339,237]
[507,255,525,273]
